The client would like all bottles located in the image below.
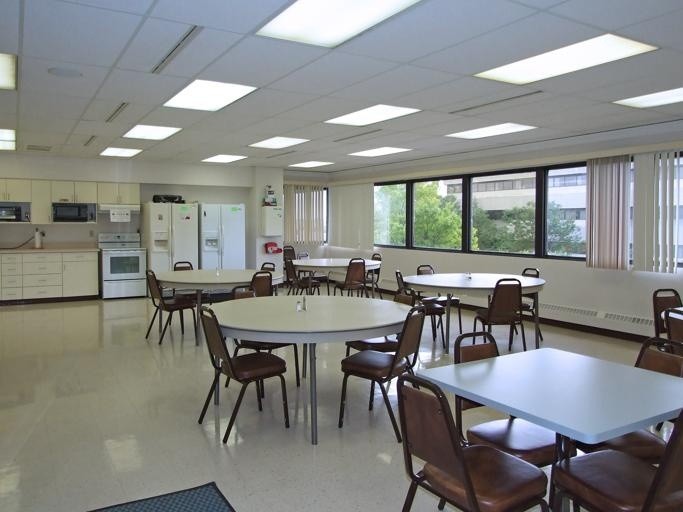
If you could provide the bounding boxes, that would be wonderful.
[302,296,306,311]
[465,272,468,280]
[265,185,275,203]
[468,272,472,281]
[296,301,302,312]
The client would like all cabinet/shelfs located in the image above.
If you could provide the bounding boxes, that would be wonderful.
[50,179,97,204]
[31,178,51,224]
[23,253,63,299]
[63,253,98,297]
[0,178,31,203]
[97,182,141,206]
[1,254,22,301]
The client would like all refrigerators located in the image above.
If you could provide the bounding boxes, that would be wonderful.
[139,201,198,271]
[198,203,245,270]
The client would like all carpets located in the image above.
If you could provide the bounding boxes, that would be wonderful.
[86,481,234,512]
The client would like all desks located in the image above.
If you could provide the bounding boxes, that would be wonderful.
[291,258,381,297]
[401,273,544,355]
[206,293,420,447]
[413,346,683,512]
[154,269,263,347]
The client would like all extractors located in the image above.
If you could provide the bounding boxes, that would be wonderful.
[98,204,141,224]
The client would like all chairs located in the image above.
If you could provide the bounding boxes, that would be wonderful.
[169,261,199,336]
[250,271,273,296]
[300,270,330,296]
[652,288,682,351]
[396,374,550,512]
[393,270,446,349]
[285,257,320,295]
[195,306,291,445]
[452,330,576,510]
[550,406,682,512]
[282,245,297,261]
[664,308,683,352]
[471,278,526,352]
[510,268,544,344]
[566,335,682,463]
[360,253,383,299]
[261,261,276,272]
[333,258,370,298]
[224,285,301,388]
[143,269,200,346]
[345,334,398,358]
[415,264,463,335]
[338,306,427,443]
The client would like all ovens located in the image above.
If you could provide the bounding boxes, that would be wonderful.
[101,251,145,280]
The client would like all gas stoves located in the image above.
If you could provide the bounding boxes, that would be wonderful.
[100,247,145,250]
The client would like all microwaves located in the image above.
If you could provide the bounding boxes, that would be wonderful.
[0,202,31,223]
[51,203,96,221]
